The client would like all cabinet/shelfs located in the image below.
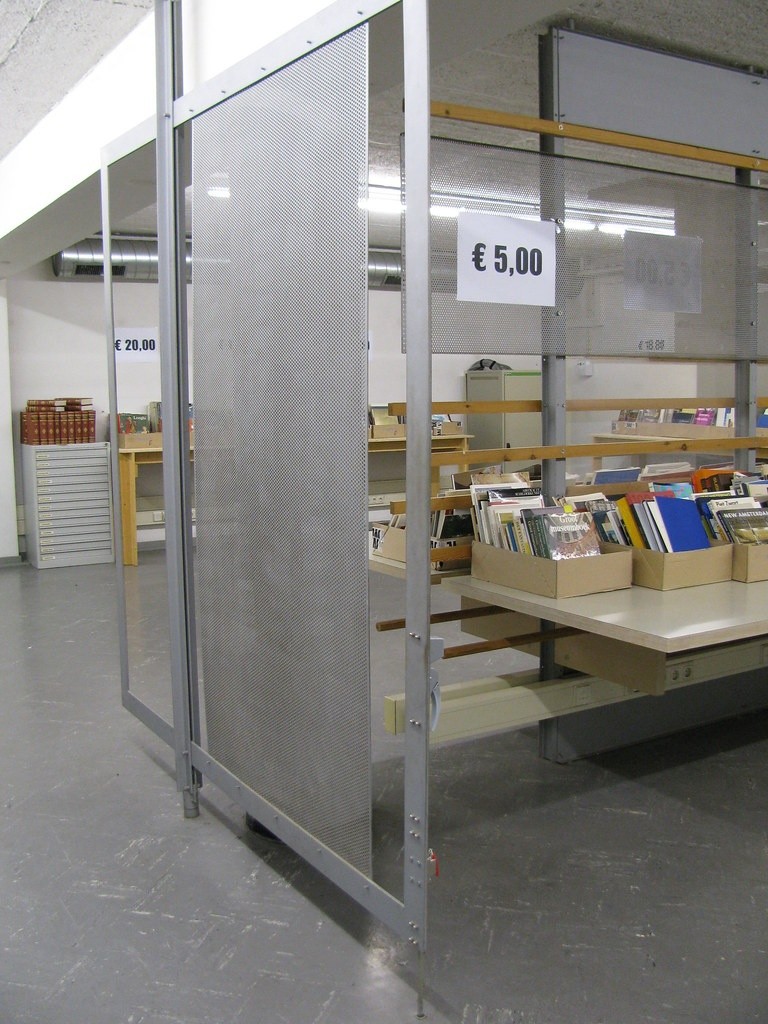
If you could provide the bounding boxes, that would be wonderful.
[21,442,115,569]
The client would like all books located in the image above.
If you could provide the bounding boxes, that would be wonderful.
[619,407,735,428]
[18,397,96,445]
[369,406,451,426]
[117,401,193,435]
[757,408,768,428]
[387,462,768,560]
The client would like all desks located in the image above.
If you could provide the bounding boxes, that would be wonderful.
[440,576,768,654]
[368,435,474,497]
[118,448,164,566]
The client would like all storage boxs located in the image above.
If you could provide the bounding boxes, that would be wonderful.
[372,424,405,437]
[630,537,732,591]
[732,542,768,584]
[371,522,407,563]
[118,432,161,447]
[441,420,461,435]
[471,541,633,600]
